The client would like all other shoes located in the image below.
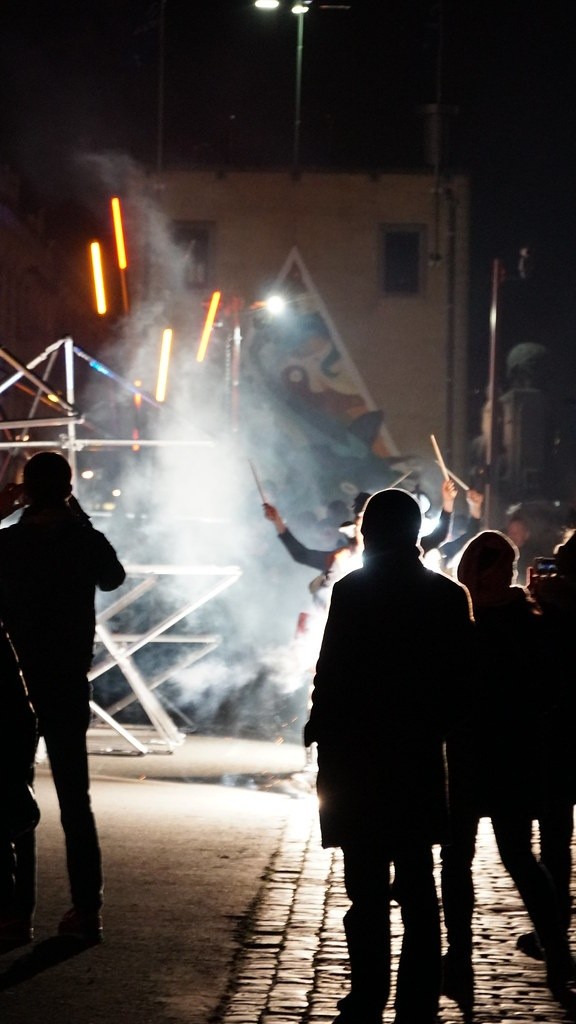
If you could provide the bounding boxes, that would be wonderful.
[65,908,104,932]
[0,920,35,941]
[546,957,575,983]
[517,931,539,948]
[442,947,473,981]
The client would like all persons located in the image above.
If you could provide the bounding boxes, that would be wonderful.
[262,478,576,1024]
[0,452,125,947]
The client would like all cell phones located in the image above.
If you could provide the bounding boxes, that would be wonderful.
[534,557,557,575]
